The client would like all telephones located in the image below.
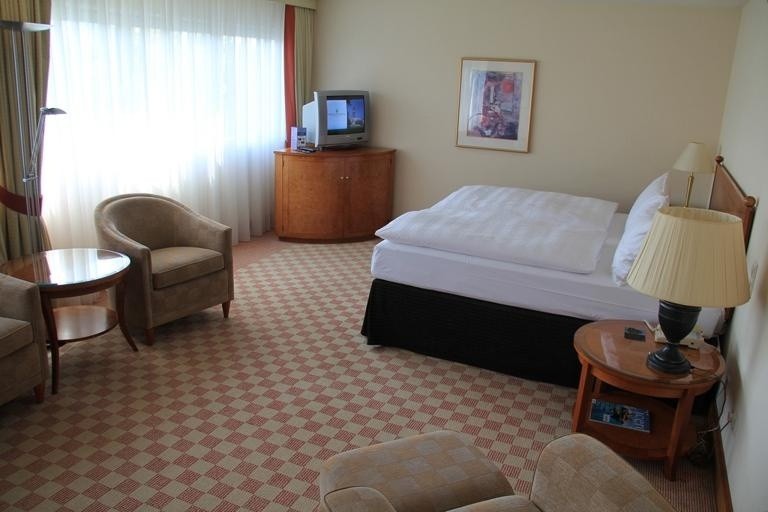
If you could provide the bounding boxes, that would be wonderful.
[645,319,705,350]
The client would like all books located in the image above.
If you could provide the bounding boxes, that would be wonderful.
[588,397,651,434]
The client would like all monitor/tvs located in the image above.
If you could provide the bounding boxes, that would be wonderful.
[302,90,370,151]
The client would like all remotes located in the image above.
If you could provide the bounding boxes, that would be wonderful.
[300,148,316,152]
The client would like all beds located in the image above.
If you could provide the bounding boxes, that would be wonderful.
[360,155,756,417]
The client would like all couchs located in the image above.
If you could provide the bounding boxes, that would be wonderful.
[93,191,234,346]
[1,275,49,411]
[318,429,680,512]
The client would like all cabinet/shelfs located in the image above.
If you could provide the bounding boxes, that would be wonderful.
[275,145,399,244]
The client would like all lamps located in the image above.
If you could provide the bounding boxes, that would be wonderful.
[672,140,717,207]
[624,205,755,378]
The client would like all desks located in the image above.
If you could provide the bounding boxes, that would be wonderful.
[568,318,727,483]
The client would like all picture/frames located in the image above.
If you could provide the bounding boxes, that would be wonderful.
[455,57,538,155]
